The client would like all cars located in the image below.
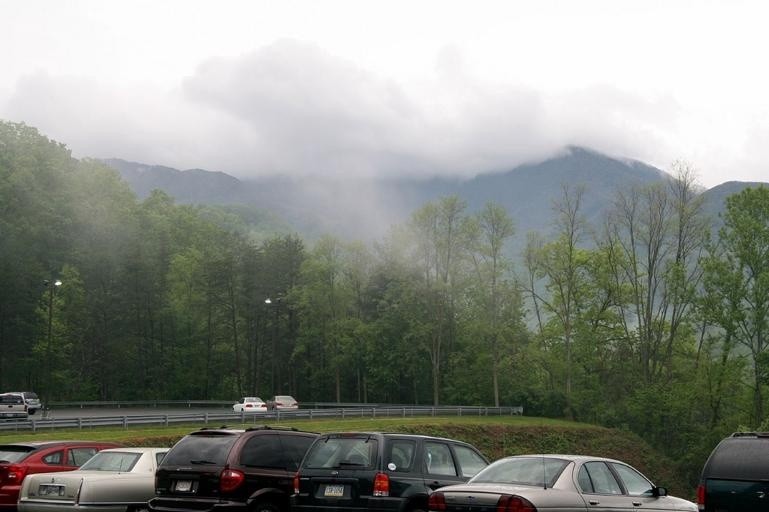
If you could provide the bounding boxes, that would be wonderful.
[0,391,41,419]
[232,395,298,412]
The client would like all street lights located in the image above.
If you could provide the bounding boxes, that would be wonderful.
[263,293,283,396]
[40,278,62,417]
